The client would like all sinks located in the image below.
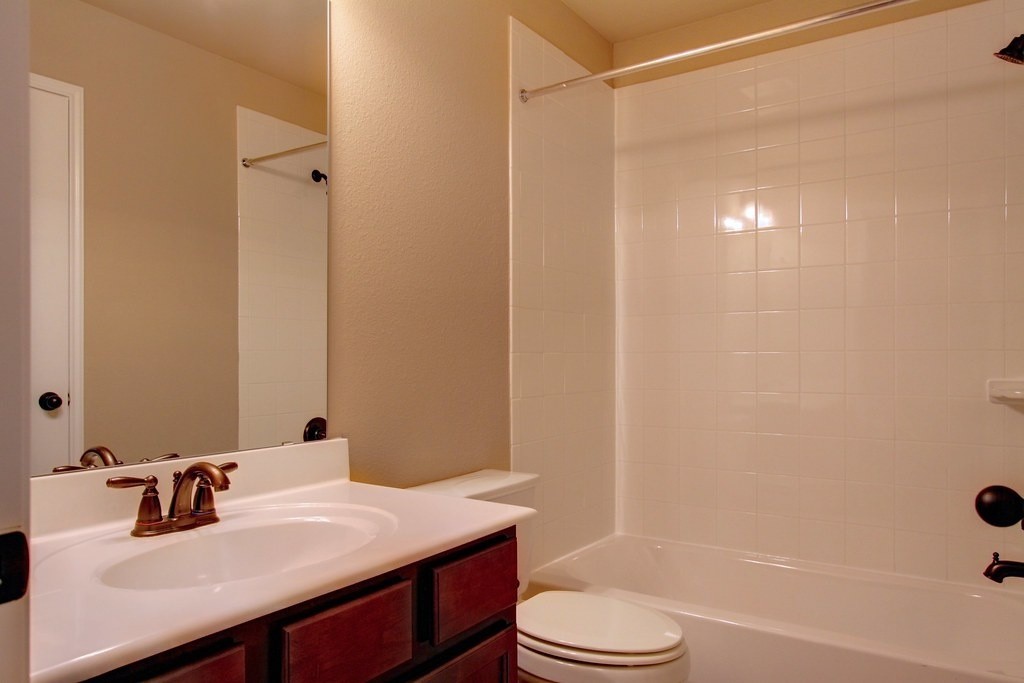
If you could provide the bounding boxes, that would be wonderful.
[94,505,380,592]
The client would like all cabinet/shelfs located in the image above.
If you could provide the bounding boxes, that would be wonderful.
[80,524,522,682]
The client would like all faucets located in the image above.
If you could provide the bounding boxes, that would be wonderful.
[972,483,1024,585]
[103,461,239,537]
[51,443,181,473]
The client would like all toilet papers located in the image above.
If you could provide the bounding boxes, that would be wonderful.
[515,567,531,596]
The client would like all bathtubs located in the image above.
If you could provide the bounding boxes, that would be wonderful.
[528,532,1024,682]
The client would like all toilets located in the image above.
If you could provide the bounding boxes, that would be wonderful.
[514,589,692,682]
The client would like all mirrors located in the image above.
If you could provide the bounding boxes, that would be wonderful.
[25,1,330,479]
